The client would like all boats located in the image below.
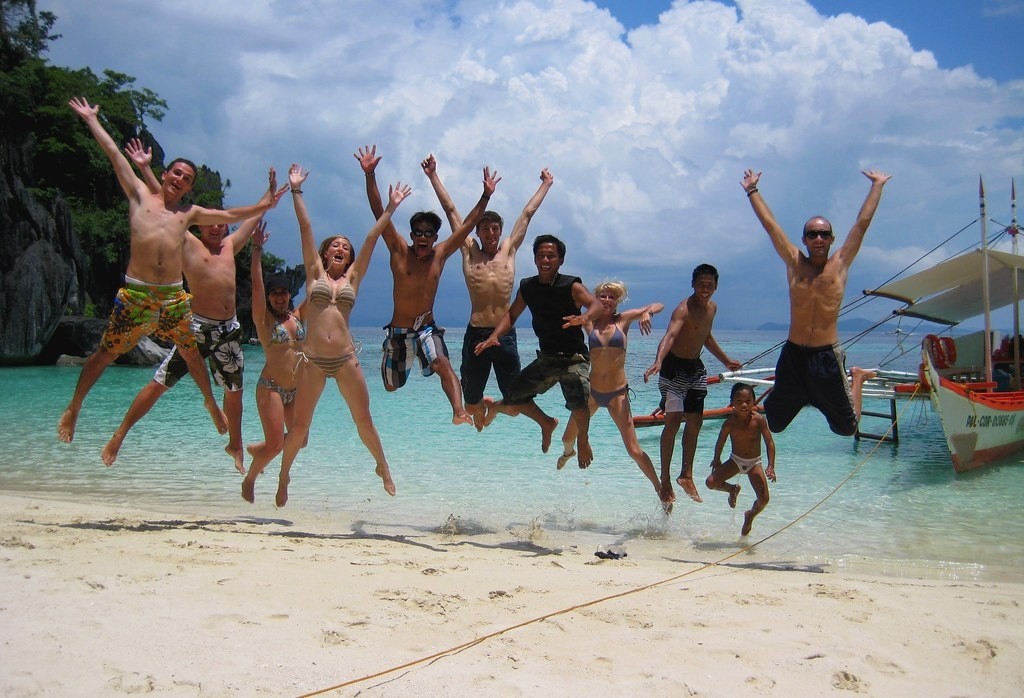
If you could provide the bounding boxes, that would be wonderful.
[633,171,1024,472]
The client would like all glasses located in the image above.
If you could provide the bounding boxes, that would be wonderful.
[804,229,832,240]
[413,230,434,237]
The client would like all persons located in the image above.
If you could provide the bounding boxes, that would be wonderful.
[352,144,502,428]
[100,138,288,476]
[644,262,741,505]
[242,220,311,504]
[555,280,673,512]
[475,235,603,470]
[738,167,893,435]
[275,163,412,508]
[421,153,554,434]
[58,93,276,446]
[705,383,778,538]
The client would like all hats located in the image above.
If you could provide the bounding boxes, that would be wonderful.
[267,277,290,295]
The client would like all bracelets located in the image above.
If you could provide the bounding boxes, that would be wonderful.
[745,185,758,196]
[482,194,490,200]
[365,172,375,175]
[253,244,263,250]
[644,308,653,318]
[291,187,303,193]
